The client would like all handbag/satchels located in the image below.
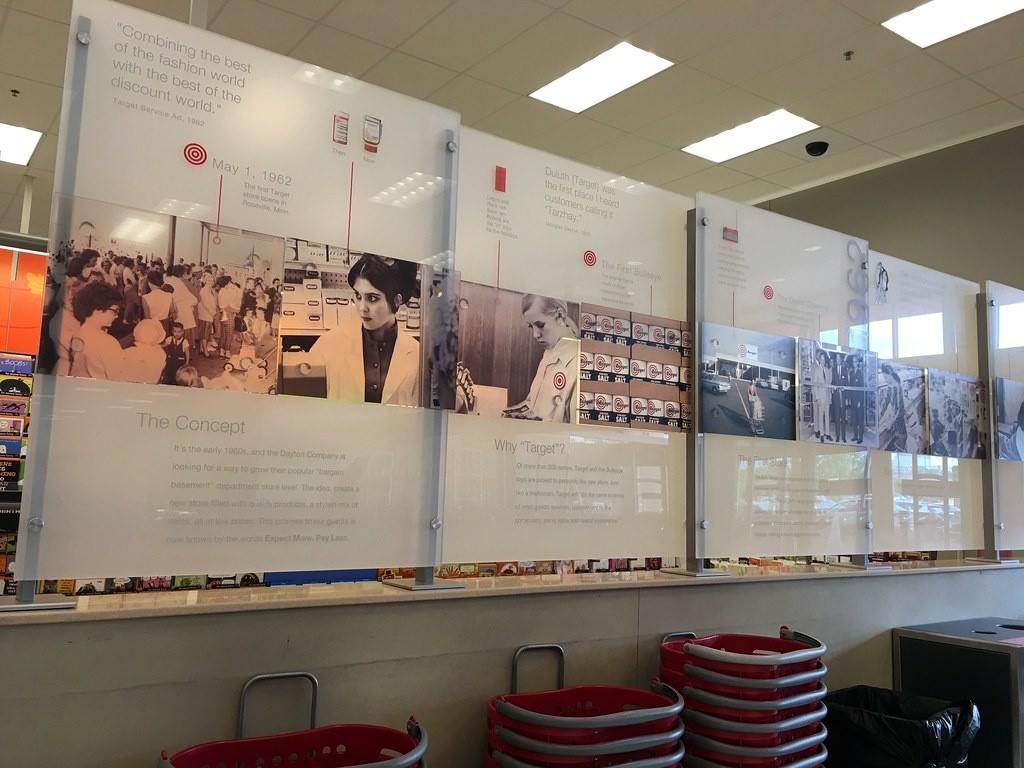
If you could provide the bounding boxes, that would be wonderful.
[234,312,247,332]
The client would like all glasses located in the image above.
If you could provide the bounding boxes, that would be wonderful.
[107,309,120,315]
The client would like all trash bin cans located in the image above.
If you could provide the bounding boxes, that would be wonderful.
[886,613,1024,768]
[813,684,980,768]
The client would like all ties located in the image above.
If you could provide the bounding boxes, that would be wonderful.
[823,368,825,379]
[838,365,841,379]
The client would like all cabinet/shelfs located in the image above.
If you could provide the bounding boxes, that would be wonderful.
[276,262,420,403]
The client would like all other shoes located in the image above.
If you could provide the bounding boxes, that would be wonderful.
[851,438,858,441]
[827,436,833,441]
[836,438,839,442]
[821,436,824,442]
[857,441,863,444]
[815,432,819,438]
[842,439,846,443]
[190,340,230,361]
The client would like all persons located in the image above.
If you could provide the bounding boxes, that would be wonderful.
[747,379,757,419]
[502,294,579,423]
[38,238,283,386]
[307,252,420,407]
[809,352,1024,462]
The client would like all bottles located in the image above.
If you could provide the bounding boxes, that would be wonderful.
[398,297,421,330]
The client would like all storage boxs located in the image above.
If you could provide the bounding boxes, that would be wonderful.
[280,237,365,331]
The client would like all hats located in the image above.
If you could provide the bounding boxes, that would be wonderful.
[134,318,165,345]
